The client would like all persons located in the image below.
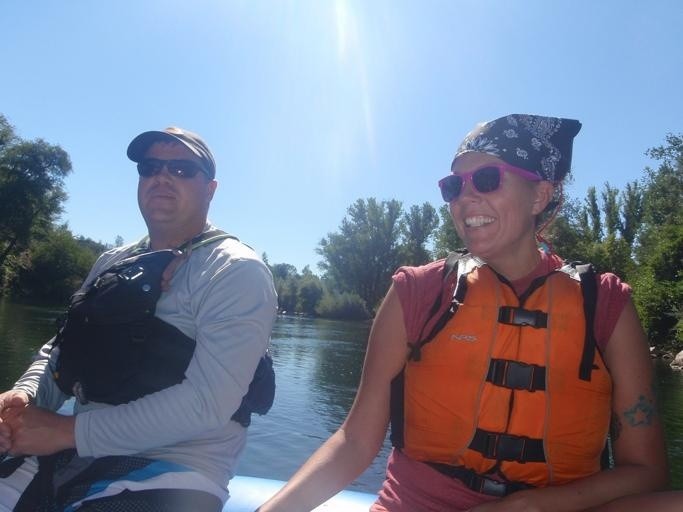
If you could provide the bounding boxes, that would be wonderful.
[253,113,683,511]
[0,126,279,512]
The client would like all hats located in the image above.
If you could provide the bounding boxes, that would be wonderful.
[125,126,216,177]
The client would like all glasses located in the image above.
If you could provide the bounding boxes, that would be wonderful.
[136,158,209,180]
[437,163,543,202]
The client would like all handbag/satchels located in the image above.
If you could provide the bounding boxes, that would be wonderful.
[55,227,237,396]
